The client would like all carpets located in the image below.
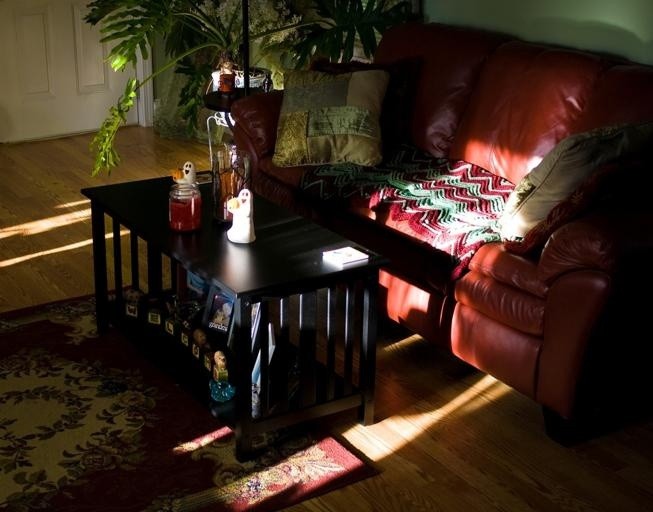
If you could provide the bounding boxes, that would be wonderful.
[2,282,368,510]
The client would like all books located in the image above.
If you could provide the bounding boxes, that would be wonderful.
[322,256,368,269]
[321,246,368,265]
[186,269,275,397]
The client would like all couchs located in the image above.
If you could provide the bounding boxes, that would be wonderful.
[228,20,651,450]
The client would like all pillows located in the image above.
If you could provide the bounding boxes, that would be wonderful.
[270,53,426,168]
[487,120,652,255]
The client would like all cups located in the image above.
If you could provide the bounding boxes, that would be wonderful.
[211,141,252,223]
